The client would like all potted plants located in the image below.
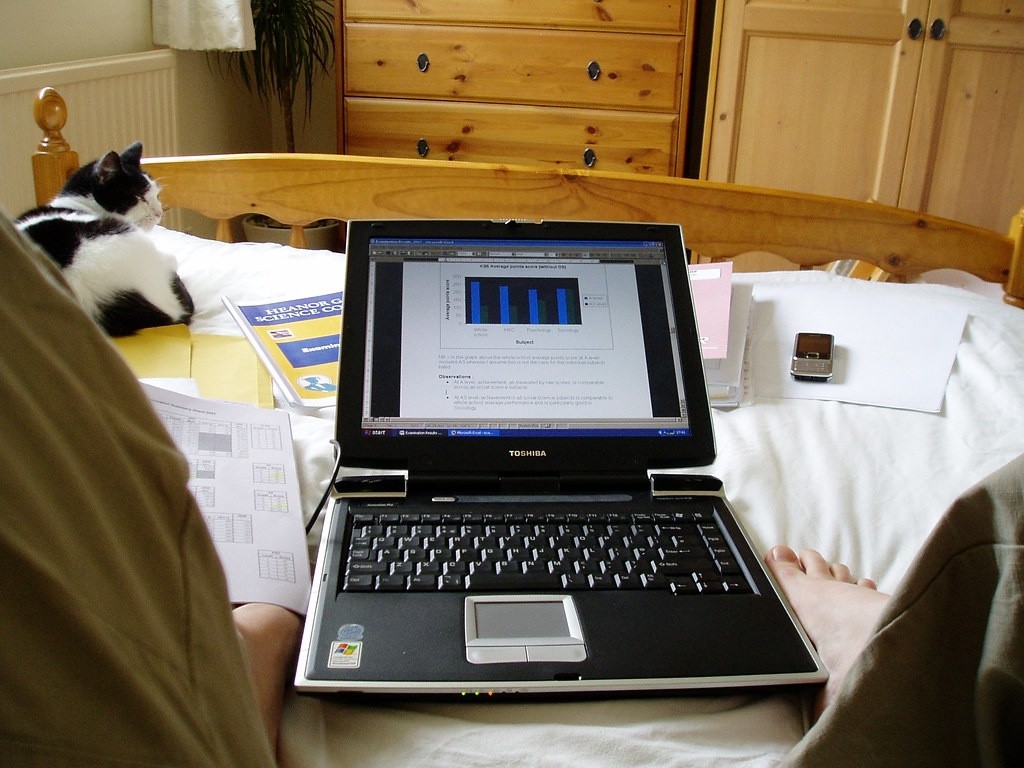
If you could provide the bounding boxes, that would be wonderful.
[206,0,335,153]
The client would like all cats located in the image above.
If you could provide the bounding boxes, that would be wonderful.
[12,141,195,338]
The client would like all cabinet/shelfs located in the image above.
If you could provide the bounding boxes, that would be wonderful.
[335,0,696,177]
[690,0,1024,283]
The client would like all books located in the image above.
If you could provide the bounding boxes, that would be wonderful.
[686,261,755,409]
[221,289,343,408]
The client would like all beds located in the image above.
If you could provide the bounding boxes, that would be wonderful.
[29,83,1024,768]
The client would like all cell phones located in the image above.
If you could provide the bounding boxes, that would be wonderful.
[791,332,834,380]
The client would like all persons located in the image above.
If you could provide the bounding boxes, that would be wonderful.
[0,214,1024,767]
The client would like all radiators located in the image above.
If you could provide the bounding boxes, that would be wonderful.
[0,49,178,219]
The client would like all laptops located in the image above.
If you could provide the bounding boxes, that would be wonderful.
[293,219,830,707]
